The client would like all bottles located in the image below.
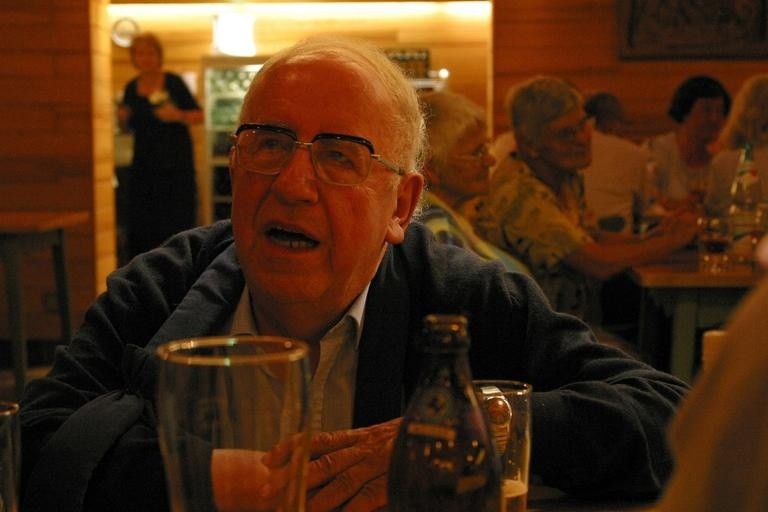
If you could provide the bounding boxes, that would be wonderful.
[726,140,766,268]
[385,313,503,512]
[0,400,22,512]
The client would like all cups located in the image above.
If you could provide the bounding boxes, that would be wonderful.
[695,214,732,275]
[471,378,535,512]
[154,332,316,512]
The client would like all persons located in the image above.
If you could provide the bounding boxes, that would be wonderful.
[415,73,767,509]
[0,33,696,510]
[113,32,207,263]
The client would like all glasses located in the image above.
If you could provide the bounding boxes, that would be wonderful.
[235,122,407,188]
[548,117,596,139]
[456,138,491,162]
